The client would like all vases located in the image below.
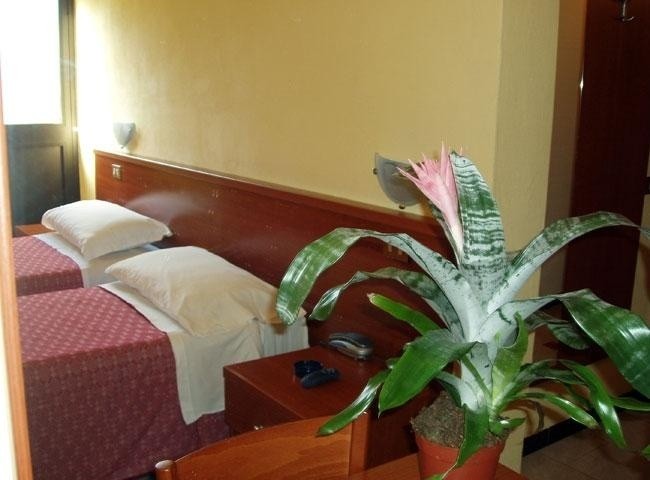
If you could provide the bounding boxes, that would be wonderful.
[415,430,505,480]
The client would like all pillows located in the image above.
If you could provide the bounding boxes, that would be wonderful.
[40,199,173,261]
[103,245,308,336]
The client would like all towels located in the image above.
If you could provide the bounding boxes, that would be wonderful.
[31,232,159,288]
[97,281,310,426]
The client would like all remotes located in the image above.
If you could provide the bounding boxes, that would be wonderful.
[300,366,340,388]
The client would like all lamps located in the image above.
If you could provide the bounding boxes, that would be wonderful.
[373,152,430,209]
[113,122,136,149]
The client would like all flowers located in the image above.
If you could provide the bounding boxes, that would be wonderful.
[274,142,650,480]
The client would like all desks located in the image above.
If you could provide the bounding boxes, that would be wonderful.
[341,453,531,480]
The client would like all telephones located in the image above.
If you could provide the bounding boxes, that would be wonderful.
[328,334,374,359]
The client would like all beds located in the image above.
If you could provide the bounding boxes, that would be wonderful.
[14,236,159,296]
[17,286,309,480]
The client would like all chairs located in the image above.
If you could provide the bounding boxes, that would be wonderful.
[156,412,368,480]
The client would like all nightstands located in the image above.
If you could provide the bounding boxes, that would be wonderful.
[223,346,436,470]
[14,223,55,236]
